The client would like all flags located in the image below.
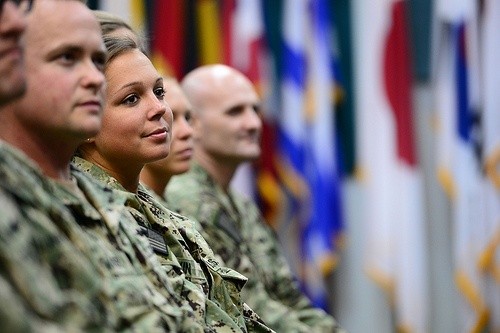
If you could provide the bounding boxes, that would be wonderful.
[85,0,500,333]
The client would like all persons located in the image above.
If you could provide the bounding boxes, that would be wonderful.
[0,0,349,333]
